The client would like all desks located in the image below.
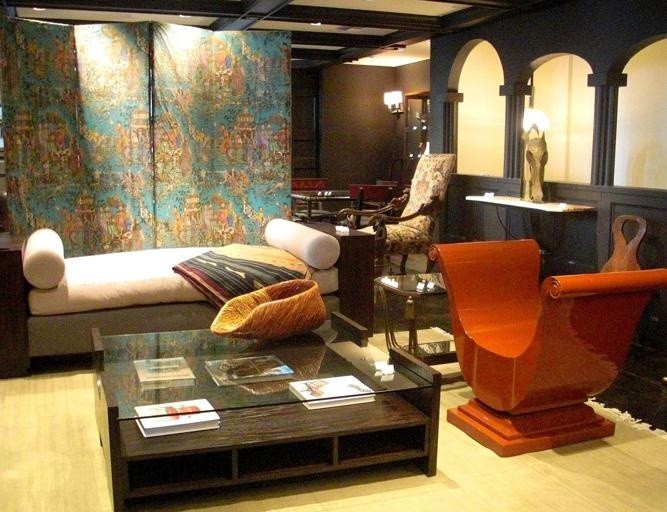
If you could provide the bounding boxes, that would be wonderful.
[374,272,464,386]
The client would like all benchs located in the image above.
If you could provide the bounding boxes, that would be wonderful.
[27,245,343,373]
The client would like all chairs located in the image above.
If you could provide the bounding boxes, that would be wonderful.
[332,153,454,281]
[290,177,401,229]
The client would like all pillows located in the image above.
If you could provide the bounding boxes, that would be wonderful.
[263,218,341,271]
[22,228,64,289]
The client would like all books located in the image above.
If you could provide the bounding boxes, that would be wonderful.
[287,375,376,410]
[132,357,196,389]
[203,353,295,387]
[132,398,221,438]
